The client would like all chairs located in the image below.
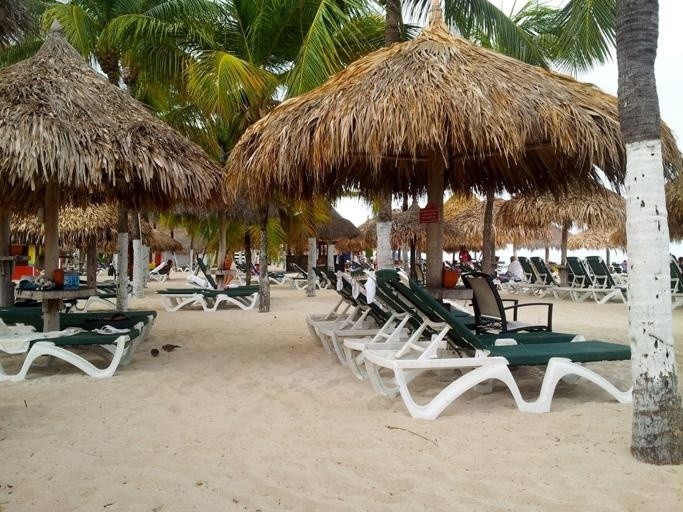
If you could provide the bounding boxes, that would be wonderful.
[279,252,681,421]
[1,256,282,386]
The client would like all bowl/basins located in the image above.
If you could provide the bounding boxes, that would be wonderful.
[443,271,461,288]
[52,271,63,288]
